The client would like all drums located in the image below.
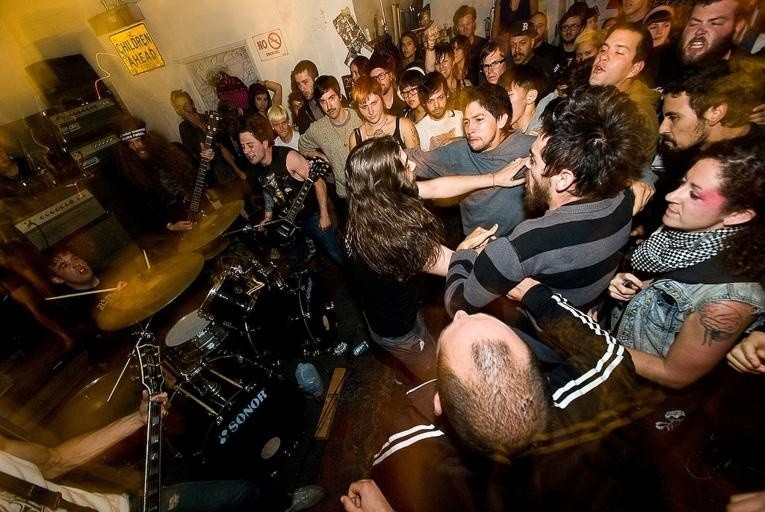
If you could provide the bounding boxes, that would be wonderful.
[287,267,340,349]
[197,250,272,333]
[157,355,306,509]
[166,311,231,363]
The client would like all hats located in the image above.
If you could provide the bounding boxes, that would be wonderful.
[509,21,539,38]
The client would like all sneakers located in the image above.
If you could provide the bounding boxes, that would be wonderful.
[288,485,326,512]
[332,340,370,360]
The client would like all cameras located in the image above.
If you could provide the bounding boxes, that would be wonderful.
[439,28,450,38]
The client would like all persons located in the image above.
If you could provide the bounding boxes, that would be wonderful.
[289,1,764,512]
[44,248,154,368]
[123,79,347,308]
[2,391,169,512]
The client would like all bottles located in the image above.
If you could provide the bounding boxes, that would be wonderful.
[296,362,327,400]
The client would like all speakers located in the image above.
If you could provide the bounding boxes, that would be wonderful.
[82,140,174,256]
[25,54,123,124]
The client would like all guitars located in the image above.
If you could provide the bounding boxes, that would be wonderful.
[255,154,331,280]
[162,109,223,239]
[131,336,166,512]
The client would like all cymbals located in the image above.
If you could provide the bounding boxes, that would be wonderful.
[177,200,245,251]
[94,251,203,329]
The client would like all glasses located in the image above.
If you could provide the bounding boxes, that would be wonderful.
[482,59,506,72]
[560,23,582,32]
[373,72,389,81]
[400,87,419,98]
